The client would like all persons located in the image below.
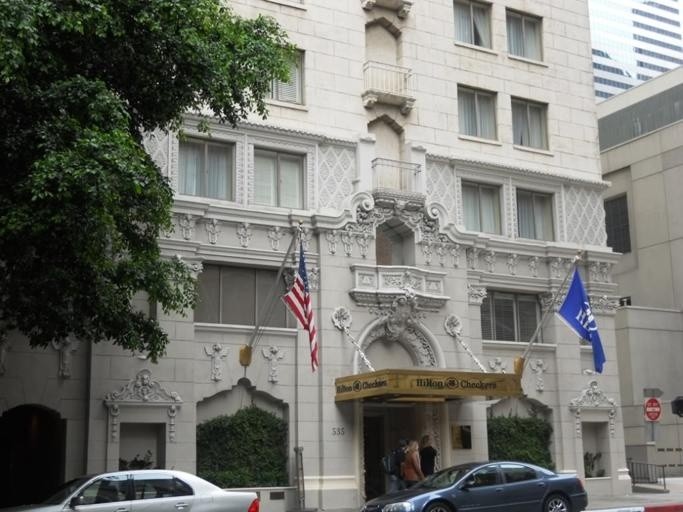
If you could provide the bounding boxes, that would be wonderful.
[474,473,488,486]
[401,440,425,487]
[383,438,408,494]
[419,435,436,478]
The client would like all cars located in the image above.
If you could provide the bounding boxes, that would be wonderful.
[357,459,590,511]
[0,467,259,512]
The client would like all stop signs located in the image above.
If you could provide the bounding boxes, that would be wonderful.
[645,397,662,421]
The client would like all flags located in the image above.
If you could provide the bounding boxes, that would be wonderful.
[554,269,606,374]
[277,239,320,373]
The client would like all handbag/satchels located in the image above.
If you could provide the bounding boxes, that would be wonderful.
[400,463,405,477]
[382,454,396,473]
